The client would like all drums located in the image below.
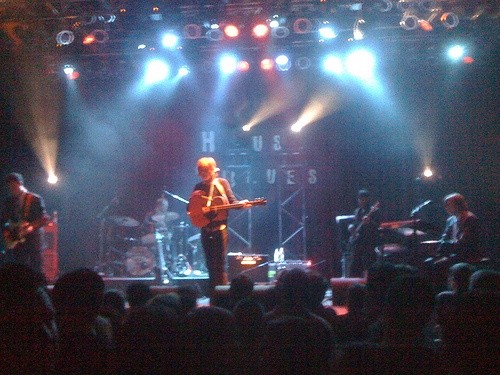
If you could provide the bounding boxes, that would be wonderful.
[122,246,158,276]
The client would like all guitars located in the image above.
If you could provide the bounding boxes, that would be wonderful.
[190,190,269,229]
[0,216,51,251]
[346,201,381,247]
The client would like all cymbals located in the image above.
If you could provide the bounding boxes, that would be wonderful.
[150,211,180,223]
[140,231,173,243]
[108,215,139,228]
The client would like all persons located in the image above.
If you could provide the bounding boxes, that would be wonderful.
[0,173,52,285]
[0,190,500,375]
[187,157,251,297]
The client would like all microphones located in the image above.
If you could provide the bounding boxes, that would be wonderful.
[410,200,432,218]
[161,186,166,199]
